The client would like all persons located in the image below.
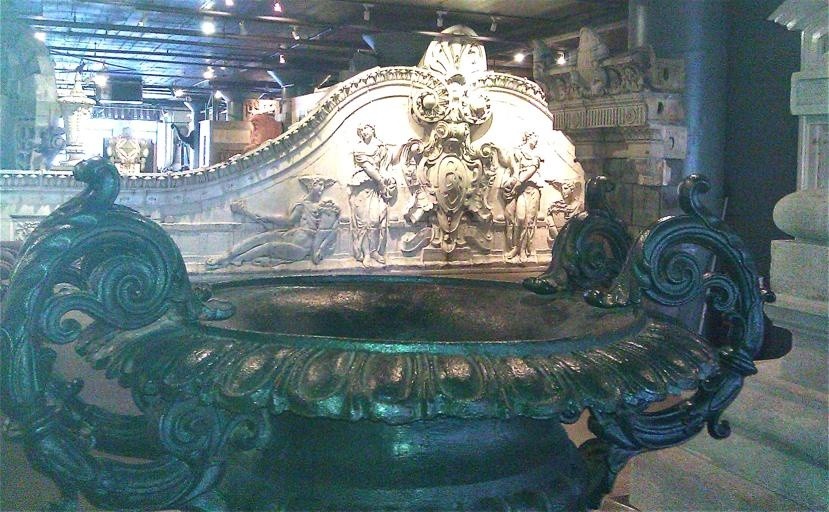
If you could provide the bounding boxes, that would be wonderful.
[546,183,587,247]
[206,175,340,267]
[491,131,545,262]
[348,122,406,268]
